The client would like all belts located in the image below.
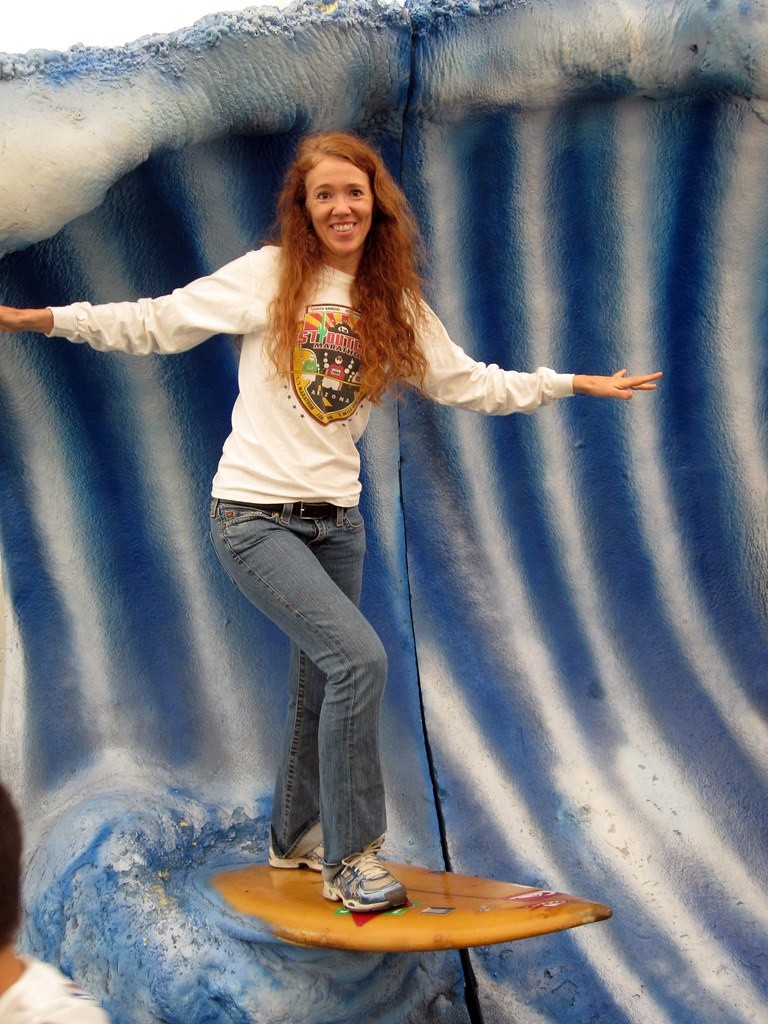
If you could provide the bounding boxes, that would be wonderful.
[212,496,352,522]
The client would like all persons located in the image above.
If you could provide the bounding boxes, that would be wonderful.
[0,132,664,909]
[0,784,114,1024]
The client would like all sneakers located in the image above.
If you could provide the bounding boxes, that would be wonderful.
[268,837,325,872]
[323,832,407,912]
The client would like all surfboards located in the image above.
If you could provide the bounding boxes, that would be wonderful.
[213,855,615,952]
